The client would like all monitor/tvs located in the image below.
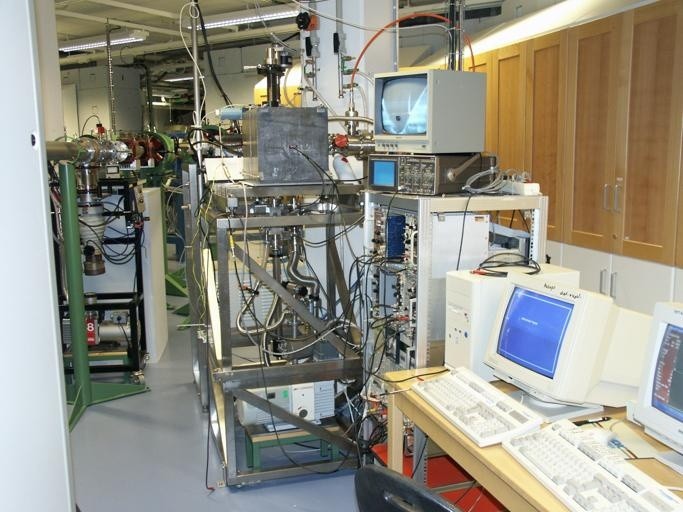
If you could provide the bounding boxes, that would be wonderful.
[483,269,652,423]
[633,300,683,474]
[374,69,486,153]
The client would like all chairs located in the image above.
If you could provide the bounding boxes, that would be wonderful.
[354,459,462,512]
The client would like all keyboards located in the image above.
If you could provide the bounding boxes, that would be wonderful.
[410,366,541,448]
[502,418,683,512]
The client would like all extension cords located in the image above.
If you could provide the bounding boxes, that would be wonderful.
[502,178,541,196]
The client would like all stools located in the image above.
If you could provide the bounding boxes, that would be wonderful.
[245,419,341,469]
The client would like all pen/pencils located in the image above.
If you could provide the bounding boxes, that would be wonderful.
[573,417,608,428]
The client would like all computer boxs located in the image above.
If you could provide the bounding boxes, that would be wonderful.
[445,263,580,382]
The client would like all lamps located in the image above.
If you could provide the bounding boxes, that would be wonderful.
[181,3,302,31]
[58,29,149,52]
[162,72,205,82]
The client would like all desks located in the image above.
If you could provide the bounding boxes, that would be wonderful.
[383,364,683,512]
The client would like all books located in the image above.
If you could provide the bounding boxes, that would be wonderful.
[580,418,657,461]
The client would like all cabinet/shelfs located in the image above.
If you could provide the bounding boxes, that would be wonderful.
[569,3,683,271]
[439,51,492,154]
[491,31,569,246]
